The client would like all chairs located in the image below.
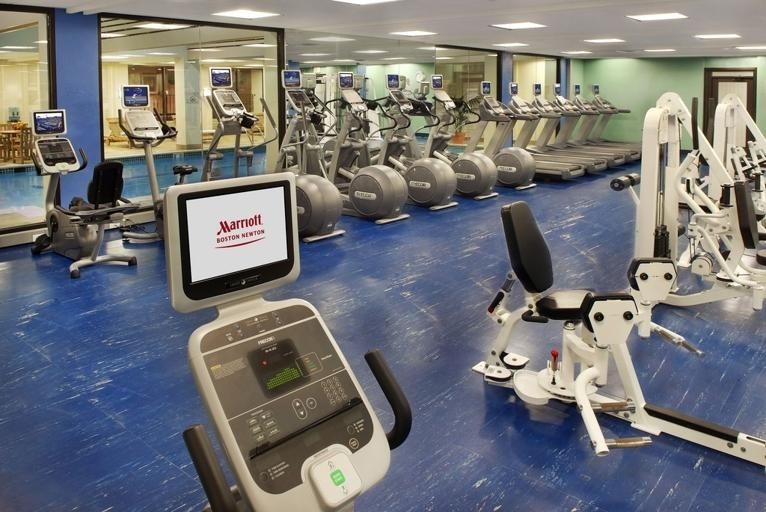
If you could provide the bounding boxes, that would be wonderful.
[247,112,264,134]
[0,122,34,164]
[107,117,130,144]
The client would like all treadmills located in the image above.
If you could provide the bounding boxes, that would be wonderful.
[454,83,646,182]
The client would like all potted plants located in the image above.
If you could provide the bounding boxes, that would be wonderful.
[451,94,484,144]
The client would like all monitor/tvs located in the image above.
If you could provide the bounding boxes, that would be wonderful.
[121,84,150,108]
[281,70,303,89]
[164,171,301,314]
[32,109,67,136]
[431,74,443,89]
[508,81,600,95]
[338,71,354,90]
[209,67,232,88]
[386,73,400,90]
[480,81,492,96]
